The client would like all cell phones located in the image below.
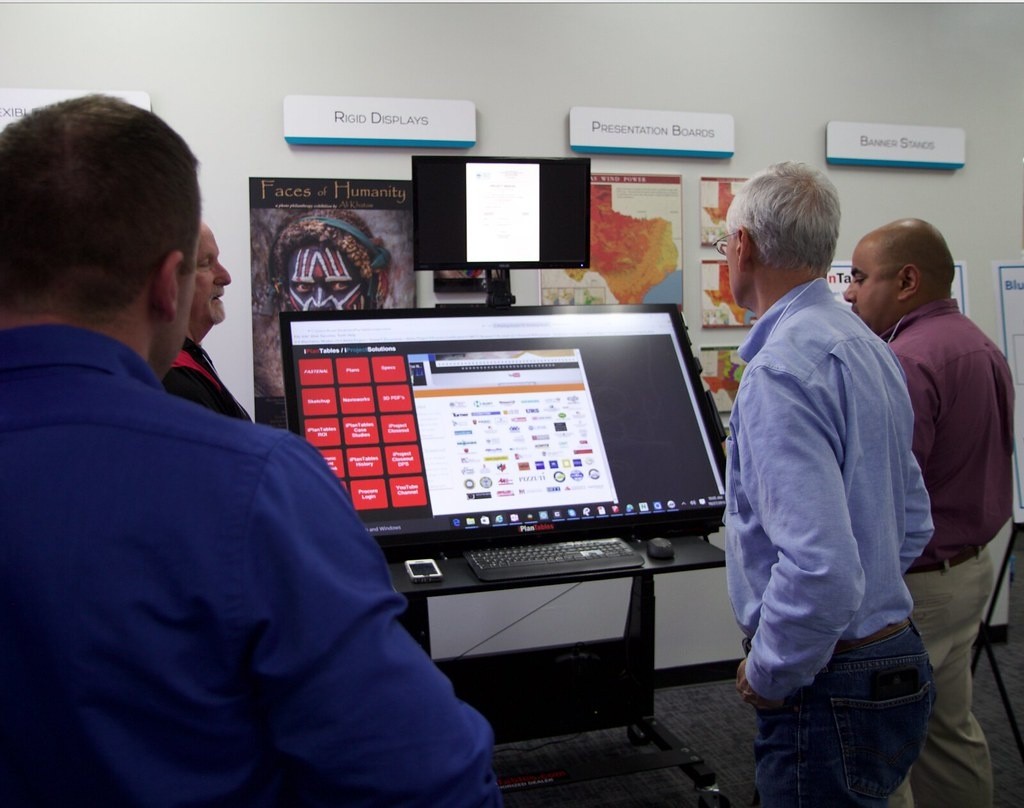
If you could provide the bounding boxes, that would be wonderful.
[405,558,443,583]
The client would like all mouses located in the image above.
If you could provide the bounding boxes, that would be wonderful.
[648,538,675,560]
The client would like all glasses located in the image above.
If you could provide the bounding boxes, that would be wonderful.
[712,231,739,256]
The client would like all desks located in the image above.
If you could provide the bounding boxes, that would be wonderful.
[383,535,732,808]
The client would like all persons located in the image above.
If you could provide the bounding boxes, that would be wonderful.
[845,218,1015,808]
[161,222,252,425]
[271,209,391,312]
[0,94,501,808]
[711,163,936,808]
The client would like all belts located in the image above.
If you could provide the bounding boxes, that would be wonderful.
[904,540,991,574]
[744,613,912,655]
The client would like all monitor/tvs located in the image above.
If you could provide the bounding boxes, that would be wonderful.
[279,303,731,564]
[410,156,590,271]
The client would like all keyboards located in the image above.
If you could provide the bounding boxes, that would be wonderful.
[464,537,645,580]
